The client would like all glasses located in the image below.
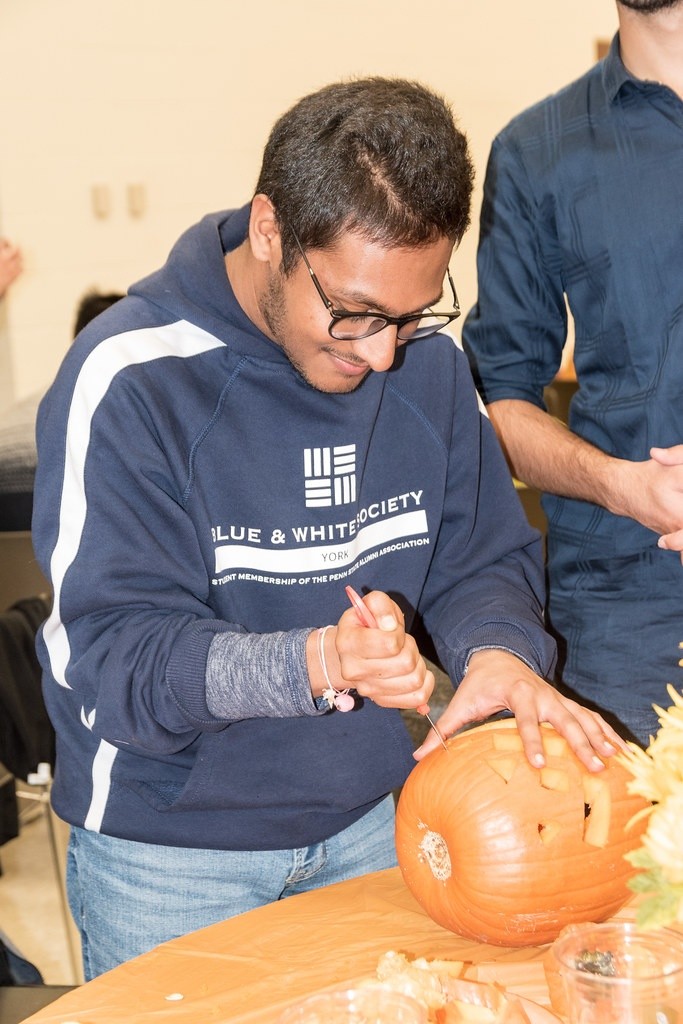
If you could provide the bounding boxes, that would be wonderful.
[286,222,461,341]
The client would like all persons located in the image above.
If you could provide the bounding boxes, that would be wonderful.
[27,79,626,985]
[461,0,683,748]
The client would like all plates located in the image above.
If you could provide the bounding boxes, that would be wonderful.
[282,980,562,1024]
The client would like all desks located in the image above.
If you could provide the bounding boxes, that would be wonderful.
[20,866,683,1024]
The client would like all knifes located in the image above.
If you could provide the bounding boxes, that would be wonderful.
[344,584,448,751]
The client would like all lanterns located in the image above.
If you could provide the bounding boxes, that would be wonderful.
[396,716,655,951]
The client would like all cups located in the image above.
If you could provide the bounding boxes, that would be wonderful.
[552,922,683,1024]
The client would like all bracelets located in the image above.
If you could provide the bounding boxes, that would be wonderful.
[318,623,356,713]
